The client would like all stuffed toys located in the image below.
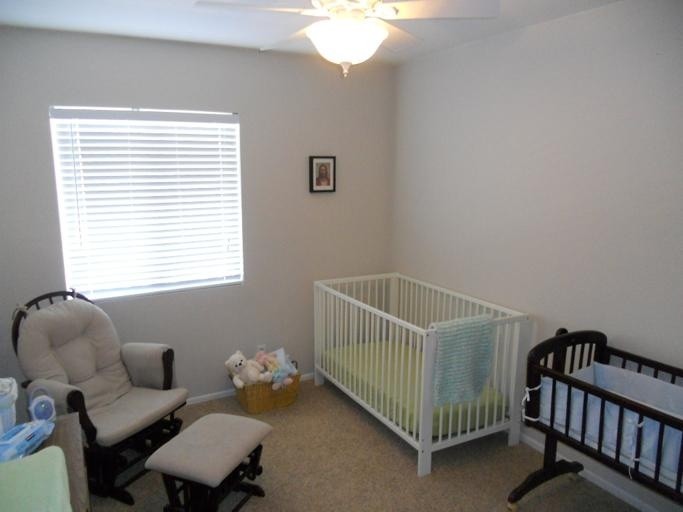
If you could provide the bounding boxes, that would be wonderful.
[254,348,298,389]
[223,350,272,389]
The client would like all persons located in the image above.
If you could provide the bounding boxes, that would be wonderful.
[315,162,330,186]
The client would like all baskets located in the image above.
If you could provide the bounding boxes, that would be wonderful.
[229,359,300,413]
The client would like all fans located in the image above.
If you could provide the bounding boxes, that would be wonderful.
[181,1,511,54]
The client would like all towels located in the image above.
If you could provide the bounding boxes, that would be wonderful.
[428,310,500,404]
[0,443,76,511]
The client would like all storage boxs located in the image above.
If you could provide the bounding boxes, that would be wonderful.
[234,370,302,414]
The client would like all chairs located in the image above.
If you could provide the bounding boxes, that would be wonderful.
[8,283,189,506]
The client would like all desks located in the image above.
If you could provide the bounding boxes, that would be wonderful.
[0,409,90,512]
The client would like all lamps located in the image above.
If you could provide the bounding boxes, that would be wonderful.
[305,15,391,76]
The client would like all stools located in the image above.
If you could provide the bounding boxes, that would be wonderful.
[142,411,273,512]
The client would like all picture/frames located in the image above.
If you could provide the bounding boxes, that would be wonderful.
[307,154,338,194]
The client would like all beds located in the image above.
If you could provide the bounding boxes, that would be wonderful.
[310,270,535,478]
[505,327,682,512]
